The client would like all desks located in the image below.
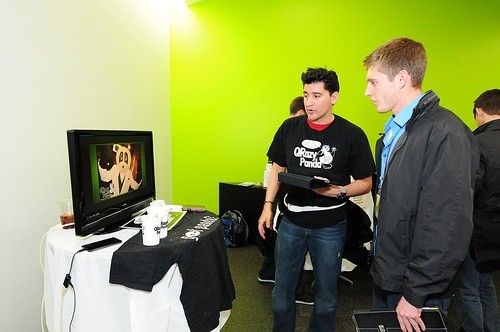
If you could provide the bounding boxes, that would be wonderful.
[43,203,236,332]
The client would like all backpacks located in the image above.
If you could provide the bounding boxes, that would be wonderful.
[221,209,249,248]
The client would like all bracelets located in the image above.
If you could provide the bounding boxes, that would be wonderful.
[264,200,273,205]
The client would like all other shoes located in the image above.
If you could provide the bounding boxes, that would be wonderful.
[295,292,315,305]
[258,269,277,283]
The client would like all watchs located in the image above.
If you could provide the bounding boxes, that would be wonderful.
[336,185,347,200]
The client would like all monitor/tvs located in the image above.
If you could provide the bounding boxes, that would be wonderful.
[66,129,155,237]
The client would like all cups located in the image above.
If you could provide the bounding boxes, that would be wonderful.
[140,215,161,246]
[148,205,170,239]
[57,201,74,229]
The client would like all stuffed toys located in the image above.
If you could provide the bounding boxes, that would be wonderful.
[97,144,143,198]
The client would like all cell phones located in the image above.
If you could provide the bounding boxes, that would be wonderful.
[82,237,121,252]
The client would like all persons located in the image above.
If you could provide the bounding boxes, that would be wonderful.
[256,66,377,332]
[257,95,315,305]
[344,198,372,295]
[469,88,500,332]
[361,36,480,332]
